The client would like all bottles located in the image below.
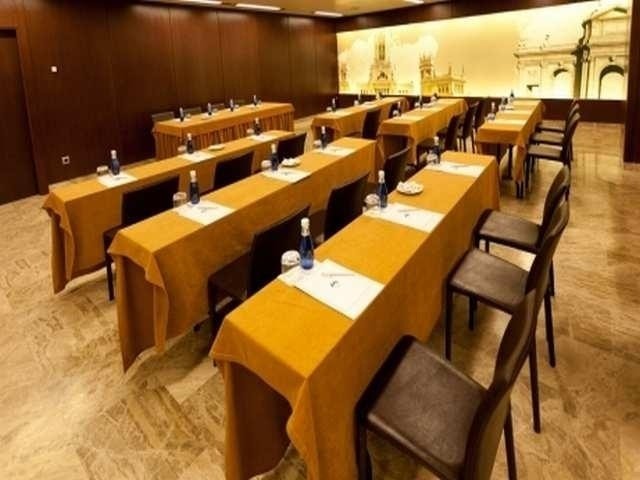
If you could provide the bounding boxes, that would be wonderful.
[378,170,388,208]
[253,95,257,106]
[321,125,327,150]
[490,101,496,120]
[208,103,212,115]
[300,218,314,269]
[110,150,120,174]
[255,118,261,135]
[359,90,363,104]
[187,132,194,153]
[418,96,423,109]
[270,144,279,170]
[179,108,184,122]
[434,136,441,163]
[398,102,402,117]
[332,99,337,112]
[230,100,234,112]
[189,171,200,204]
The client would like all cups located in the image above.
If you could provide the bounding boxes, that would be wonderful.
[326,107,332,116]
[313,140,322,153]
[427,153,437,171]
[261,161,272,175]
[394,111,399,120]
[246,129,254,142]
[172,192,187,212]
[177,146,187,161]
[281,250,303,287]
[366,193,381,216]
[488,113,495,124]
[97,166,110,183]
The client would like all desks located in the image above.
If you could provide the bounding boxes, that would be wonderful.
[40,128,296,295]
